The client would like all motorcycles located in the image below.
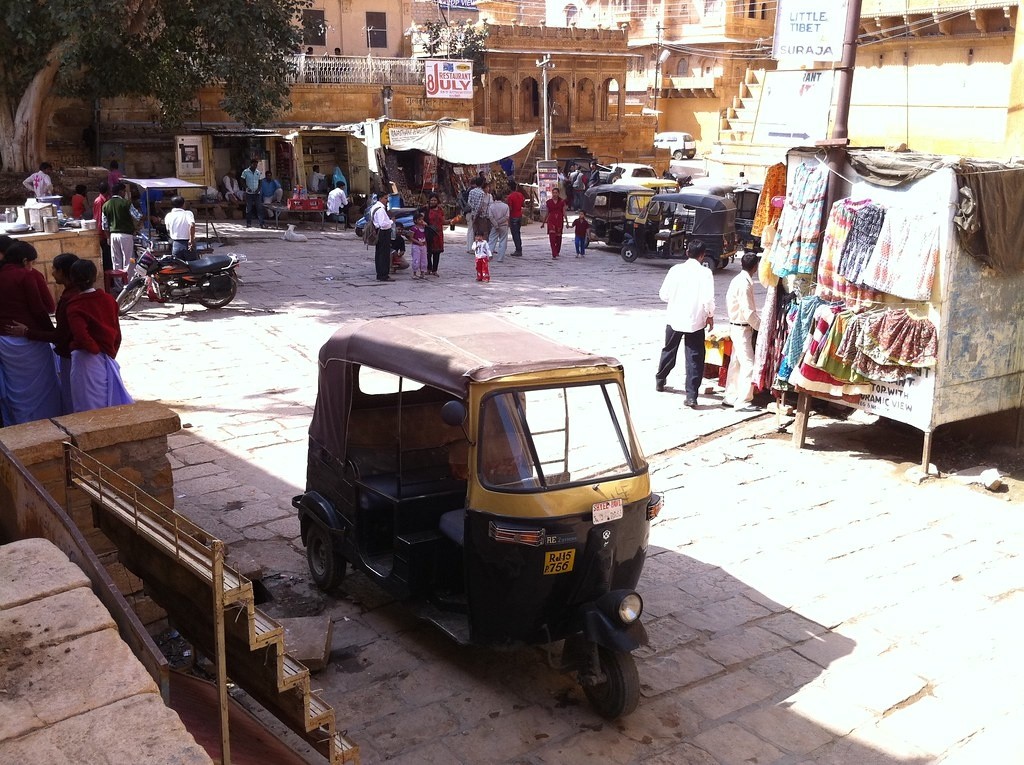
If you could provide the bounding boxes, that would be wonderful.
[290,311,665,718]
[662,164,694,188]
[113,233,248,315]
[548,156,763,274]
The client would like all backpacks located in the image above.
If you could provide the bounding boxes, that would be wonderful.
[362,207,382,246]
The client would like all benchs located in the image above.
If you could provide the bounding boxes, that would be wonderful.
[360,462,570,598]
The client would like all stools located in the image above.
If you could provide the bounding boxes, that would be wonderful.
[104,269,128,296]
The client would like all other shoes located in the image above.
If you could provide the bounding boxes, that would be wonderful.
[420,273,427,279]
[384,273,395,281]
[377,277,386,282]
[656,382,665,392]
[476,278,482,281]
[412,274,418,279]
[432,270,439,277]
[684,396,699,407]
[344,224,355,228]
[511,250,522,256]
[425,269,432,275]
[552,255,557,261]
[576,252,579,257]
[735,401,759,413]
[722,400,734,408]
[260,222,268,229]
[485,278,488,283]
[247,221,251,227]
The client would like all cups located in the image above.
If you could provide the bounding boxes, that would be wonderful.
[43,217,59,233]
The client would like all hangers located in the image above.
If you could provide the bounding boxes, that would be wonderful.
[801,283,926,318]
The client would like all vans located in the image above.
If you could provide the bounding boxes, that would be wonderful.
[653,132,696,160]
[597,163,659,186]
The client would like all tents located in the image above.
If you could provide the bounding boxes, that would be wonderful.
[385,124,539,211]
[119,176,208,248]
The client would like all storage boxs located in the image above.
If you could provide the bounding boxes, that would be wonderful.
[287,198,324,212]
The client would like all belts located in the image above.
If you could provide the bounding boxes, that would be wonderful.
[729,320,750,328]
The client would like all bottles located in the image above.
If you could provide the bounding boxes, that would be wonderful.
[10,207,15,222]
[292,188,307,199]
[5,208,11,223]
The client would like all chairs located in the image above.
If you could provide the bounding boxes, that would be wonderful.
[654,216,683,247]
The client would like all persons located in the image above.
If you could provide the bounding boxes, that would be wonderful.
[305,47,317,82]
[221,168,240,209]
[72,184,93,220]
[327,181,355,229]
[471,230,493,282]
[370,192,396,282]
[655,172,762,410]
[261,171,283,220]
[240,158,266,228]
[540,187,591,260]
[464,171,525,262]
[308,164,328,193]
[51,252,135,415]
[333,48,350,83]
[131,197,144,229]
[389,221,410,273]
[405,214,429,279]
[94,160,158,294]
[22,161,54,198]
[164,196,198,261]
[423,192,451,276]
[332,166,347,196]
[497,157,600,212]
[0,233,64,428]
